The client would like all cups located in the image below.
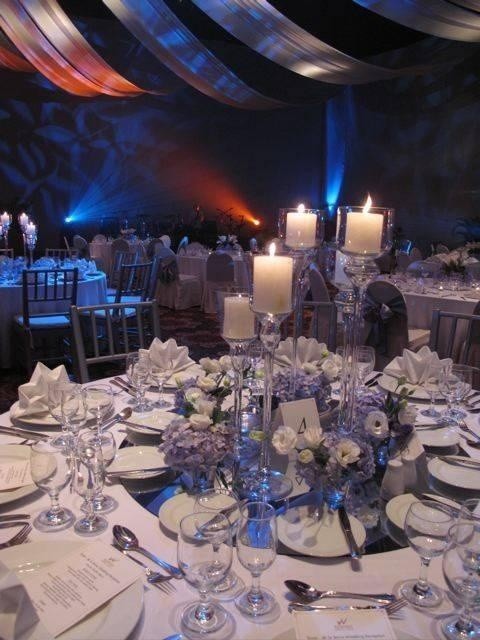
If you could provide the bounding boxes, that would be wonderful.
[146,352,175,411]
[358,346,376,393]
[193,488,246,603]
[442,522,479,640]
[233,500,279,616]
[334,347,351,396]
[79,431,120,512]
[177,510,234,636]
[125,353,152,408]
[68,446,112,534]
[46,378,83,438]
[402,501,458,608]
[446,361,474,424]
[423,365,442,419]
[131,357,155,412]
[379,459,406,503]
[80,386,112,468]
[30,437,76,532]
[439,372,463,425]
[453,498,480,613]
[401,455,419,489]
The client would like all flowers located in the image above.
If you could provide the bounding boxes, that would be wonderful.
[357,390,413,442]
[161,425,237,489]
[278,425,371,488]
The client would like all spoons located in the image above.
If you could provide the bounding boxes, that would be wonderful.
[0,425,76,454]
[281,577,398,605]
[455,418,479,442]
[110,524,186,585]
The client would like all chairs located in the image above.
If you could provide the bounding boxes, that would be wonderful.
[68,299,160,384]
[308,266,337,340]
[99,248,143,346]
[407,260,441,276]
[178,243,205,255]
[46,248,78,264]
[156,248,202,308]
[0,248,14,260]
[435,244,449,255]
[409,247,423,261]
[108,239,131,291]
[160,234,171,252]
[431,308,480,392]
[100,262,152,358]
[148,239,168,268]
[360,281,431,365]
[72,235,87,257]
[203,252,246,313]
[464,262,480,276]
[13,269,79,376]
[105,254,159,353]
[397,253,411,271]
[93,235,107,251]
[278,302,336,357]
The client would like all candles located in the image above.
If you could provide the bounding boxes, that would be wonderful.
[25,221,36,235]
[224,293,255,339]
[345,194,385,256]
[1,212,10,227]
[19,212,29,226]
[253,242,294,313]
[284,203,318,250]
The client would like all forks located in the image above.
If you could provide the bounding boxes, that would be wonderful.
[109,538,178,597]
[1,525,34,551]
[287,598,409,618]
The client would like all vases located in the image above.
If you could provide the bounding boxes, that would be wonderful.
[373,439,393,471]
[324,486,347,509]
[190,463,216,496]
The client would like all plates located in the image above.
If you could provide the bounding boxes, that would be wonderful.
[158,490,250,542]
[384,491,476,544]
[8,394,115,427]
[268,505,367,559]
[124,409,184,437]
[1,443,70,508]
[428,455,479,491]
[0,533,146,640]
[105,444,172,481]
[413,420,460,448]
[377,373,473,402]
[124,363,208,393]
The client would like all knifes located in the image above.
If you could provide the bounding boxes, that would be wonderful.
[430,451,478,470]
[405,484,480,525]
[337,503,365,561]
[0,513,31,521]
[103,463,175,480]
[114,416,166,436]
[113,374,147,402]
